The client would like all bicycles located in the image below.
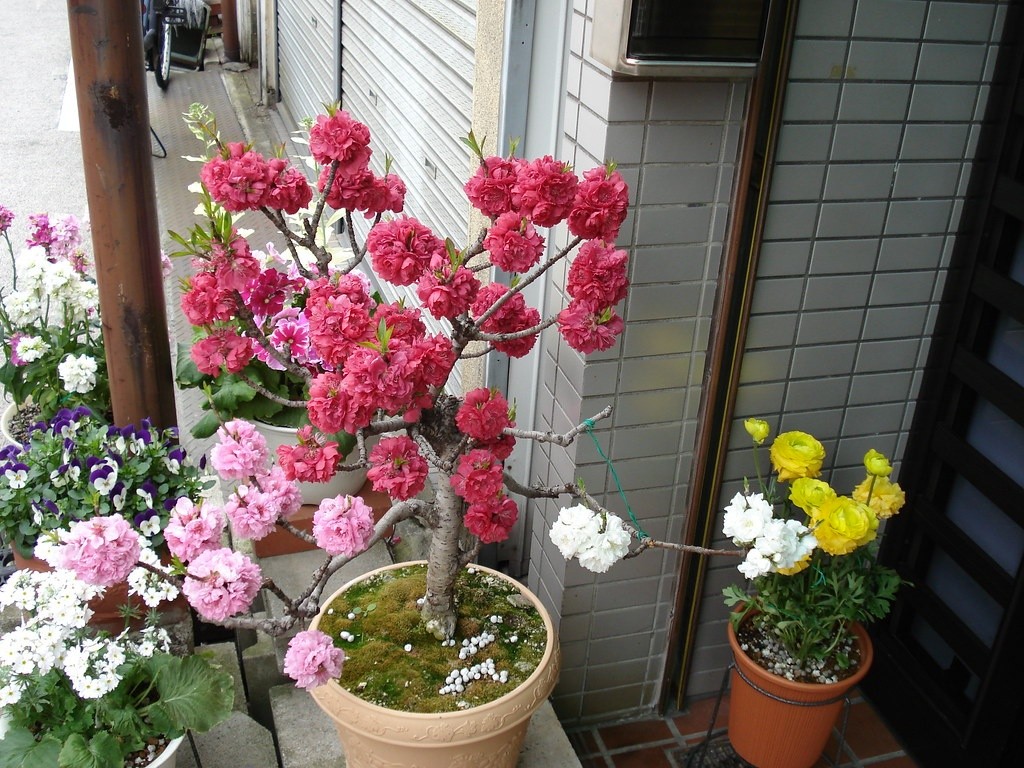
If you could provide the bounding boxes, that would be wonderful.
[143,0,188,91]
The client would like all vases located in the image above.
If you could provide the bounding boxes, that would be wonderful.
[307,560,561,768]
[8,538,191,637]
[1,384,49,448]
[728,595,872,767]
[239,414,386,507]
[144,729,186,768]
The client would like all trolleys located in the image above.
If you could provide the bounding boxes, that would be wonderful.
[169,0,213,72]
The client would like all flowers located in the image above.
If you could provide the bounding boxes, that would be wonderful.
[0,102,911,768]
